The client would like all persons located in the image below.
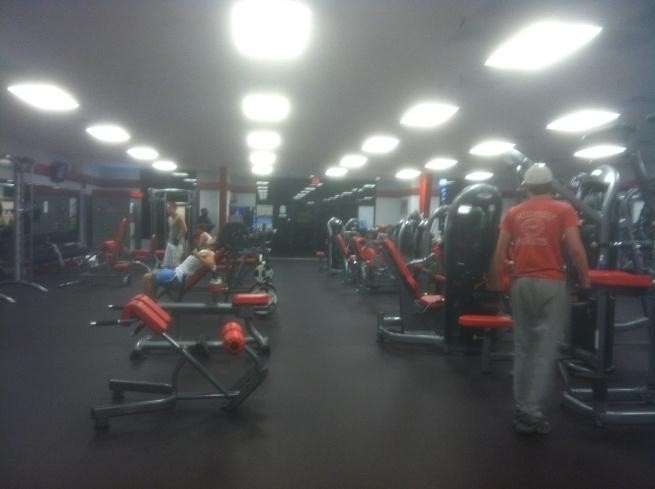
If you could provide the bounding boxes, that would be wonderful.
[196,207,215,234]
[160,200,188,268]
[194,224,215,250]
[142,235,222,302]
[493,161,593,435]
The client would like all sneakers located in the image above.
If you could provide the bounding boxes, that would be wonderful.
[512,407,552,436]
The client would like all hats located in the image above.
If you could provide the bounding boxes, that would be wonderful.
[519,161,556,186]
